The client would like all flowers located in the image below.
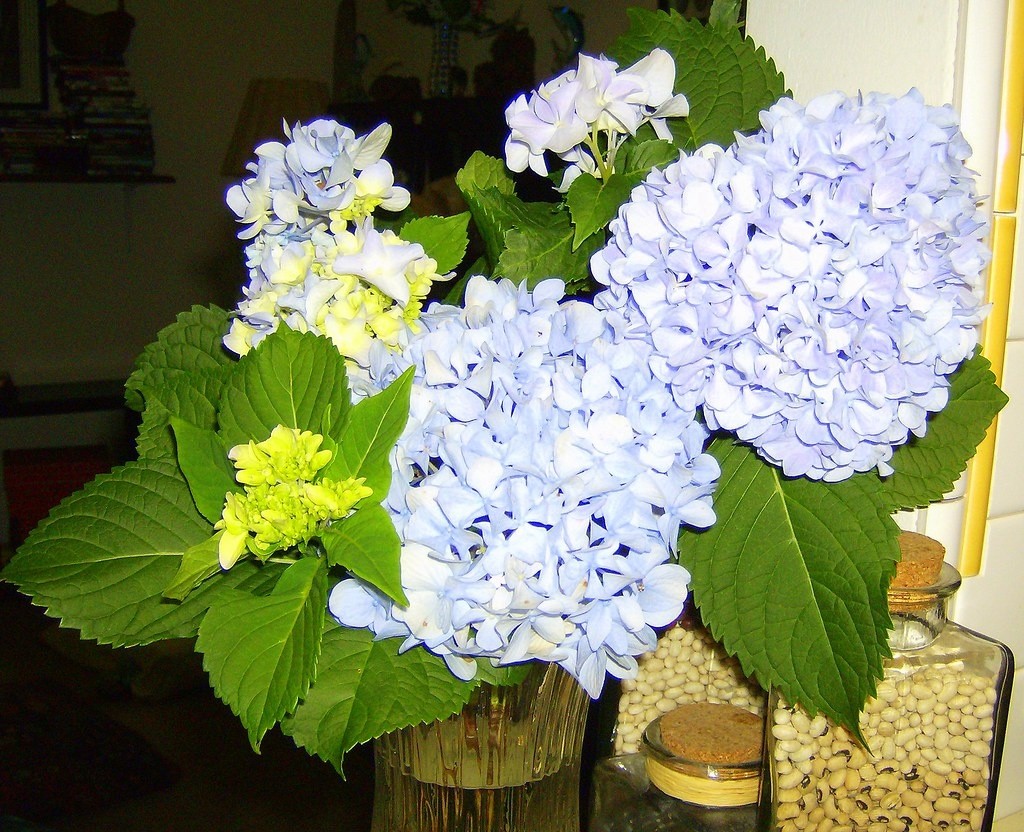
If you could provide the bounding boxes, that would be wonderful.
[0,0,1011,784]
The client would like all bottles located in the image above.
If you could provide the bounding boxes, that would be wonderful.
[589,704,767,832]
[767,531,1012,832]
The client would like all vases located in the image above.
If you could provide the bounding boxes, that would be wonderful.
[371,662,590,832]
